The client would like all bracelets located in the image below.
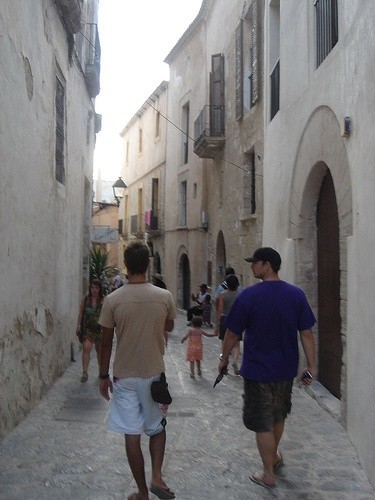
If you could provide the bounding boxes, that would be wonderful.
[219,354,229,362]
[99,373,109,379]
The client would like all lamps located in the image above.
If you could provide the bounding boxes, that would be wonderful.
[91,174,127,215]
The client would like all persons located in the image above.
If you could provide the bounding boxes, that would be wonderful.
[76,268,244,383]
[217,248,316,488]
[98,242,175,500]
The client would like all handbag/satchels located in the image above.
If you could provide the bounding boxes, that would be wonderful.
[79,328,85,344]
[151,372,172,405]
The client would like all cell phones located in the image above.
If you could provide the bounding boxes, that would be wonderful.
[301,371,314,383]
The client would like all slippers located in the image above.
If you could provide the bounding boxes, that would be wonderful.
[150,485,176,500]
[127,484,148,500]
[248,470,277,490]
[272,451,283,470]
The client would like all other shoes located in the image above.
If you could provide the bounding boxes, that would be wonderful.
[190,371,201,379]
[201,322,215,328]
[80,371,88,383]
[186,322,192,326]
[231,362,241,376]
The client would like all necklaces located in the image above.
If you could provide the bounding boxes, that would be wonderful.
[126,281,147,284]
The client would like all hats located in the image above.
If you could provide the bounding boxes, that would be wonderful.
[243,247,282,264]
